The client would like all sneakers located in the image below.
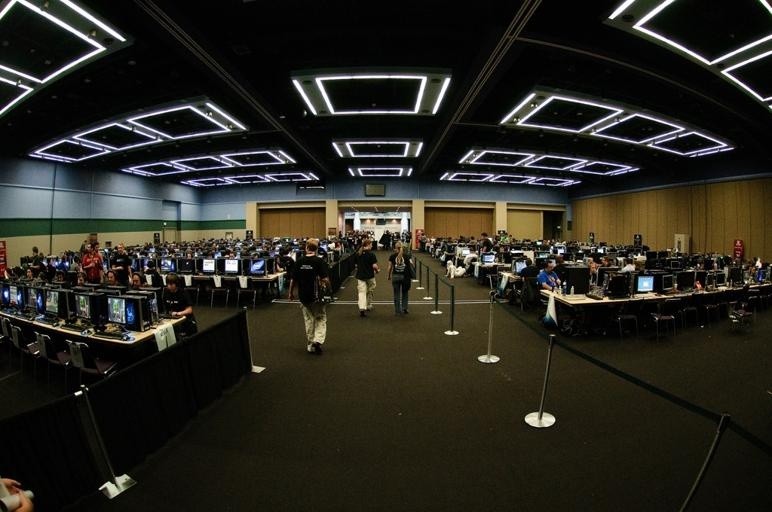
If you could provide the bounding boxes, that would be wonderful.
[307,342,322,353]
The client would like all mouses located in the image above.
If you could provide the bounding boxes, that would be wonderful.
[122,334,129,341]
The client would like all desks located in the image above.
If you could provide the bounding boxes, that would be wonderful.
[425,236,770,340]
[1,237,357,395]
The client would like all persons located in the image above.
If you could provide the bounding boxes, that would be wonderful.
[0,478,34,512]
[6,233,379,352]
[386,231,771,319]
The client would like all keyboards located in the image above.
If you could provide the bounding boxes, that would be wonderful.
[0,307,123,341]
[160,313,182,319]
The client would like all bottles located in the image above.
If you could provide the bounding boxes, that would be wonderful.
[677,238,681,251]
[711,278,733,291]
[552,280,574,297]
[591,285,604,298]
[714,262,717,270]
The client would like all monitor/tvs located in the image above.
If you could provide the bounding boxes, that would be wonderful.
[0,275,163,332]
[45,238,356,276]
[430,238,772,293]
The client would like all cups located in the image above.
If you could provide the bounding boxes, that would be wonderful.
[673,283,678,289]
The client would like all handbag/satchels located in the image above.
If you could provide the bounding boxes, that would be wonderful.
[313,273,334,304]
[408,262,416,279]
